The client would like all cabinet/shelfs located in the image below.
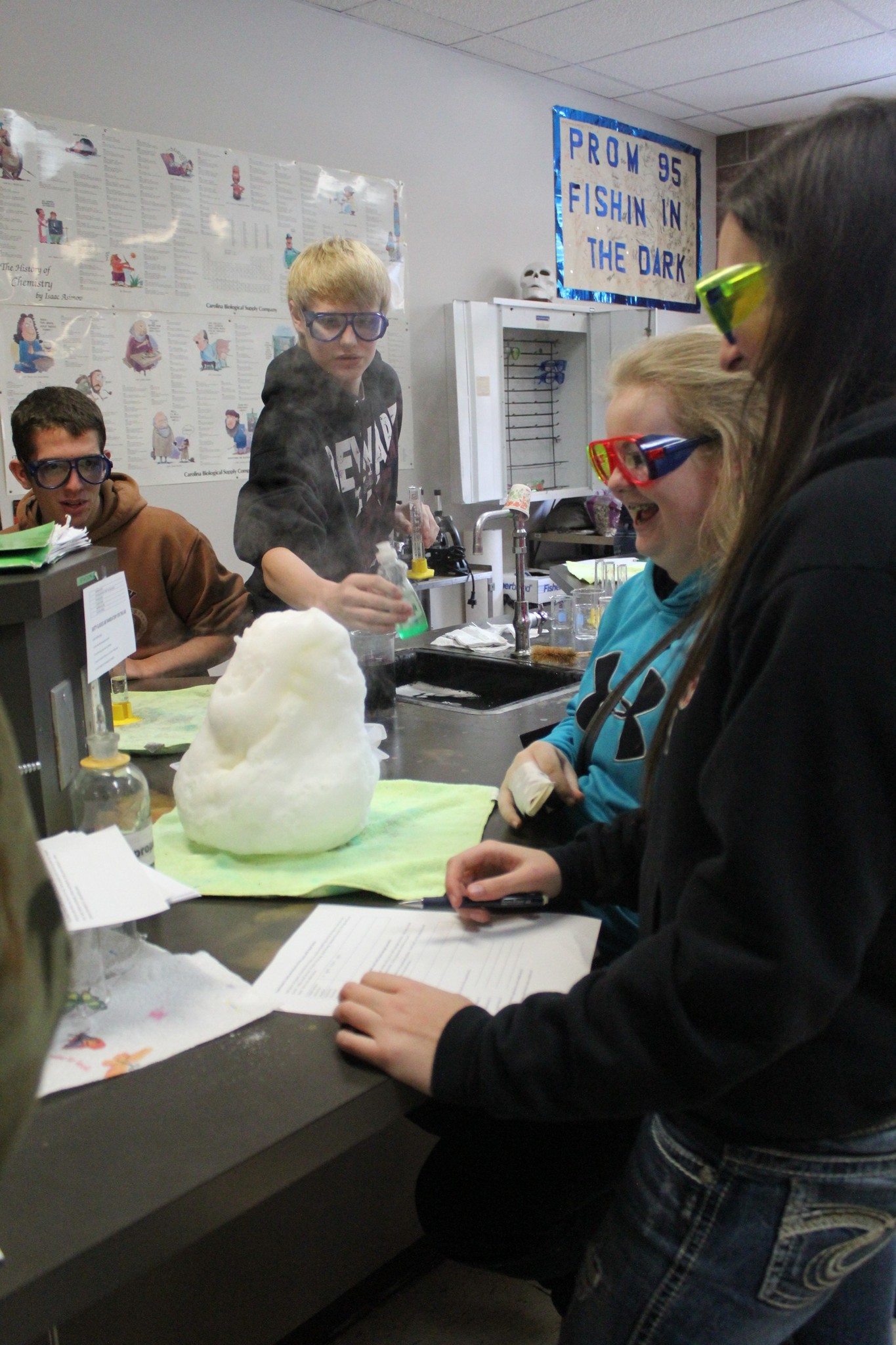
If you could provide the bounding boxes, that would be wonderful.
[444,297,659,506]
[527,532,616,569]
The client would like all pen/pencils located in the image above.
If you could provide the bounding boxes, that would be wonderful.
[397,894,552,913]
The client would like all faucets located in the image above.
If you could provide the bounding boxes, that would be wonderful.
[472,508,531,658]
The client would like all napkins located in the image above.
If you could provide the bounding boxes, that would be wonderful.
[430,621,549,654]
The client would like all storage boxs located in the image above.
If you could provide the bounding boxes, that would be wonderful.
[502,567,566,604]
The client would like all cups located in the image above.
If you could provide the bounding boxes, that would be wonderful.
[570,587,614,640]
[350,628,397,709]
[549,595,572,629]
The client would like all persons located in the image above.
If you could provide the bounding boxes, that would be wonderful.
[225,238,441,635]
[0,386,255,683]
[496,322,763,835]
[330,89,896,1345]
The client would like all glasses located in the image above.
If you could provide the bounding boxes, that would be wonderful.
[297,300,389,341]
[587,431,721,489]
[695,258,779,344]
[19,451,114,489]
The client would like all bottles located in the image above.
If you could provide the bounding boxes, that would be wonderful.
[70,731,156,868]
[375,540,428,640]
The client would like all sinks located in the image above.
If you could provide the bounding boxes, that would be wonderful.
[389,648,584,715]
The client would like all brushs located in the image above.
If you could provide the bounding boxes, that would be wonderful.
[530,646,592,666]
